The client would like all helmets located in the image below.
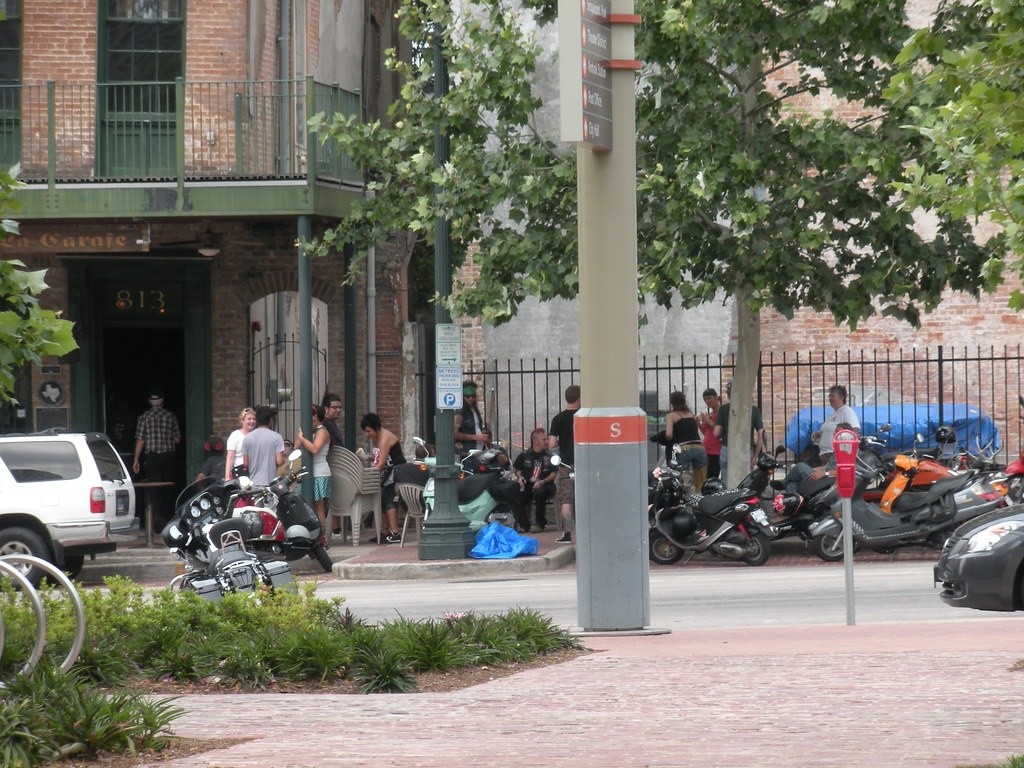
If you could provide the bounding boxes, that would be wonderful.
[672,511,697,536]
[285,525,311,542]
[701,478,726,496]
[935,427,956,443]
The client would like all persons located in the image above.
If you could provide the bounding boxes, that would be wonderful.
[131,389,181,480]
[278,439,302,477]
[453,382,491,452]
[320,394,345,534]
[225,408,257,488]
[241,406,285,509]
[811,385,860,465]
[512,428,557,534]
[665,391,709,495]
[548,385,581,543]
[771,422,859,491]
[713,380,765,482]
[196,435,226,481]
[293,405,332,545]
[361,412,406,543]
[695,388,722,477]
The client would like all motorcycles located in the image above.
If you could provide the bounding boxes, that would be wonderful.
[160,475,299,612]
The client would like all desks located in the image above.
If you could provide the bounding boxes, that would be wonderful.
[133,482,175,550]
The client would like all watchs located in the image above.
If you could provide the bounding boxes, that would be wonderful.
[825,471,829,477]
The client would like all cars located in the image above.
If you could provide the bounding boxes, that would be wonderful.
[932,501,1024,614]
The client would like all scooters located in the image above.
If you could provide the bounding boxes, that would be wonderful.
[410,435,520,532]
[548,393,1024,568]
[238,448,334,573]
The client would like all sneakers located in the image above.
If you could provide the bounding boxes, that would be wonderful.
[369,531,402,543]
[556,531,572,544]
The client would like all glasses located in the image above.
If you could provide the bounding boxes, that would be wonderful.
[243,409,251,417]
[463,394,476,398]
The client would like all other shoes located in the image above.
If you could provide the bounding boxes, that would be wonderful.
[530,525,542,532]
[770,480,785,490]
[515,527,526,532]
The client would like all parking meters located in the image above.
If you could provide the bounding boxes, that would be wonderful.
[831,429,861,625]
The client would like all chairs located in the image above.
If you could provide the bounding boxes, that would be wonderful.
[395,481,430,547]
[323,445,382,548]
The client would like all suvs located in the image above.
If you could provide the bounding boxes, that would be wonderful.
[0,425,141,591]
